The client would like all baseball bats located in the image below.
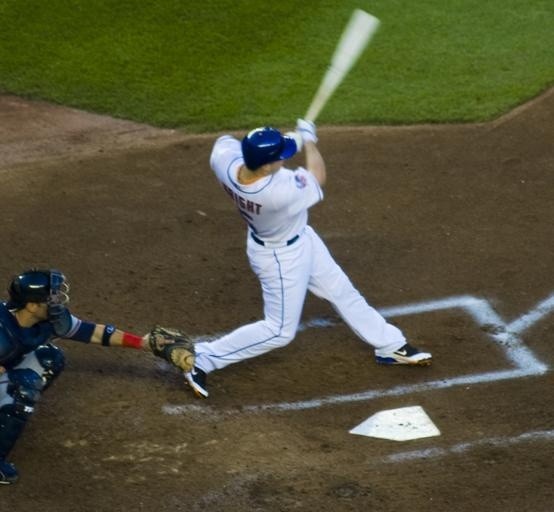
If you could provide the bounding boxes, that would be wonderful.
[303,9,381,121]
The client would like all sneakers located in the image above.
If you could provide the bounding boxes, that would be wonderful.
[0,462,19,484]
[183,366,209,399]
[375,344,433,366]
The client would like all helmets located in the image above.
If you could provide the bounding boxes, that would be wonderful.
[242,126,296,171]
[6,269,69,323]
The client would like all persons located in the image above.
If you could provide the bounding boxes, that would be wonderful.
[0,268,191,490]
[180,119,434,400]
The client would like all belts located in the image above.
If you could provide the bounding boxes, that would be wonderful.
[251,233,299,246]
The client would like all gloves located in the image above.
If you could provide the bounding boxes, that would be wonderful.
[284,119,319,153]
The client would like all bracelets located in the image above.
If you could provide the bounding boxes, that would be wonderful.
[121,330,143,350]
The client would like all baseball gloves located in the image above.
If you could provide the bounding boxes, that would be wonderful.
[149,324,196,371]
